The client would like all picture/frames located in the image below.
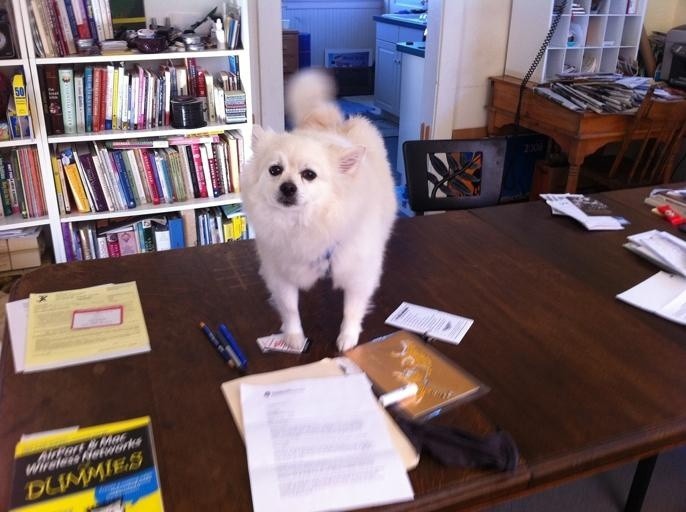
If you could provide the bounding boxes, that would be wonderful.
[324,48,373,68]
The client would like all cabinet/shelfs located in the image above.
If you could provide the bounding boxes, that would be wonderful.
[373,21,425,126]
[282,29,300,78]
[504,0,647,85]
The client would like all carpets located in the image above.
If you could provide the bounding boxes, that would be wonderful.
[285,98,376,132]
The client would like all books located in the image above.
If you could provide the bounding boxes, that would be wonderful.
[345,329,482,419]
[534,1,678,115]
[22,279,153,375]
[7,414,166,511]
[1,0,251,262]
[217,355,421,473]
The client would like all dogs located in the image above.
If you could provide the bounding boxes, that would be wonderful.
[239,65,399,352]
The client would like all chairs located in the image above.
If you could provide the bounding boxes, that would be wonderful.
[402,137,508,216]
[578,85,686,190]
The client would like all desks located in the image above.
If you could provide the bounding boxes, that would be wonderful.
[486,75,686,194]
[1,183,686,510]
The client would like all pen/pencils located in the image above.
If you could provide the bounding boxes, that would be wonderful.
[199,320,248,373]
